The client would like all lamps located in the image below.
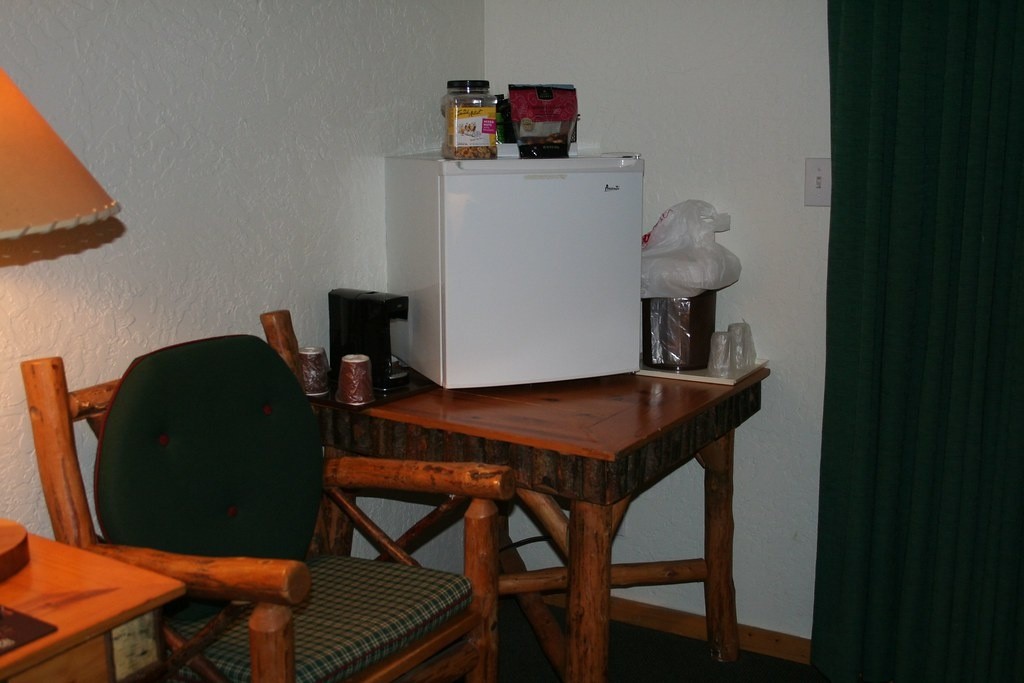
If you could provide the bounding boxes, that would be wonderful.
[0,71,122,582]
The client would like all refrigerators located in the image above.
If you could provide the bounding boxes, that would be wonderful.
[385,155,646,388]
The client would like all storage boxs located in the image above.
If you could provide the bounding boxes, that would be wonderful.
[642,289,715,367]
[509,84,577,123]
[520,143,568,160]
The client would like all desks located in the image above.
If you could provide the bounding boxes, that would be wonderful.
[319,357,762,683]
[0,518,186,683]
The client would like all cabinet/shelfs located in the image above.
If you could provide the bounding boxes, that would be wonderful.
[383,159,647,386]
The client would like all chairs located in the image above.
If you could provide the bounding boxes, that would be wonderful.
[28,313,512,683]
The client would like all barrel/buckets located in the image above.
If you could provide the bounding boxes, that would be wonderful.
[642,289,716,370]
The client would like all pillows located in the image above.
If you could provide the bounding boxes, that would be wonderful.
[94,332,324,593]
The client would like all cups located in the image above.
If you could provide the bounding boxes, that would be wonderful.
[708,333,734,378]
[729,324,753,371]
[335,354,373,407]
[300,346,330,396]
